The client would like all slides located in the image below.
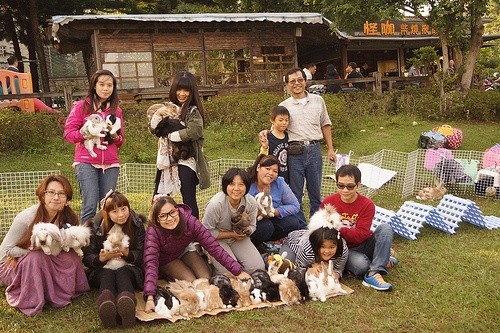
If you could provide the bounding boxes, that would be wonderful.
[34,97,60,113]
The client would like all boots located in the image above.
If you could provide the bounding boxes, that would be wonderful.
[115,291,137,328]
[97,289,117,328]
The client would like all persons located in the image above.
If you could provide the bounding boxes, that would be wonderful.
[82,190,146,328]
[202,168,268,274]
[149,71,211,221]
[5,56,19,89]
[342,62,366,93]
[242,152,301,247]
[320,164,399,291]
[258,68,337,218]
[279,227,348,281]
[302,63,323,93]
[0,175,95,315]
[408,64,420,89]
[361,63,369,78]
[142,193,253,312]
[324,65,342,94]
[259,104,290,185]
[449,60,456,75]
[64,70,125,225]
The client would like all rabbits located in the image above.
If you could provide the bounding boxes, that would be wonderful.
[413,180,447,201]
[306,202,346,240]
[230,204,251,239]
[61,225,90,258]
[143,250,348,321]
[79,111,109,159]
[253,186,278,221]
[30,221,63,256]
[145,99,198,167]
[100,224,131,271]
[102,112,123,146]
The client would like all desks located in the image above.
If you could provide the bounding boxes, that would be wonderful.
[134,89,219,105]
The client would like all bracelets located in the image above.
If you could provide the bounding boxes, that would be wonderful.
[112,135,118,139]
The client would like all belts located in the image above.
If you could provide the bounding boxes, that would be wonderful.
[297,140,319,145]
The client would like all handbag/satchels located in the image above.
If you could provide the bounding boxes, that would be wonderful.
[288,142,304,155]
[417,125,463,150]
[424,143,500,199]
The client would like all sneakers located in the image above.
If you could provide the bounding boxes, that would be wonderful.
[361,272,393,291]
[387,256,397,267]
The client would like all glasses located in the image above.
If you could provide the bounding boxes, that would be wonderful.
[44,190,67,198]
[288,78,304,83]
[158,207,178,221]
[336,182,357,191]
